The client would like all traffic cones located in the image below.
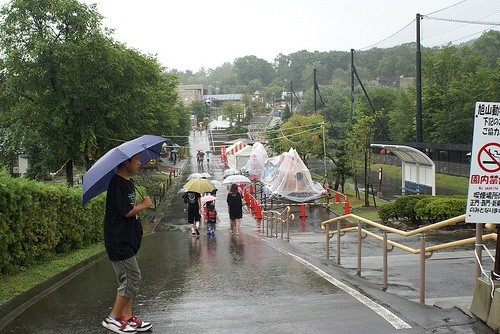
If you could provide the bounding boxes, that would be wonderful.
[298,201,308,219]
[244,183,263,219]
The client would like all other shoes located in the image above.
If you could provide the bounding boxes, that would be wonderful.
[191,232,195,237]
[195,227,200,235]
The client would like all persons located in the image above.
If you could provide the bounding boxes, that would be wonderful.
[233,174,246,198]
[196,151,209,165]
[172,153,178,160]
[182,191,201,237]
[199,177,218,238]
[101,152,154,334]
[227,184,242,234]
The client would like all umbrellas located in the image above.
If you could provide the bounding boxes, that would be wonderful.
[192,126,197,129]
[205,150,211,153]
[222,175,251,183]
[171,150,178,152]
[224,169,239,174]
[183,178,216,193]
[187,173,203,180]
[177,188,188,193]
[199,152,205,157]
[82,134,170,203]
[199,122,203,124]
[201,196,217,202]
[201,173,211,177]
[196,149,202,151]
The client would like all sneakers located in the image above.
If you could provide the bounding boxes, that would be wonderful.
[122,317,152,332]
[101,315,137,334]
